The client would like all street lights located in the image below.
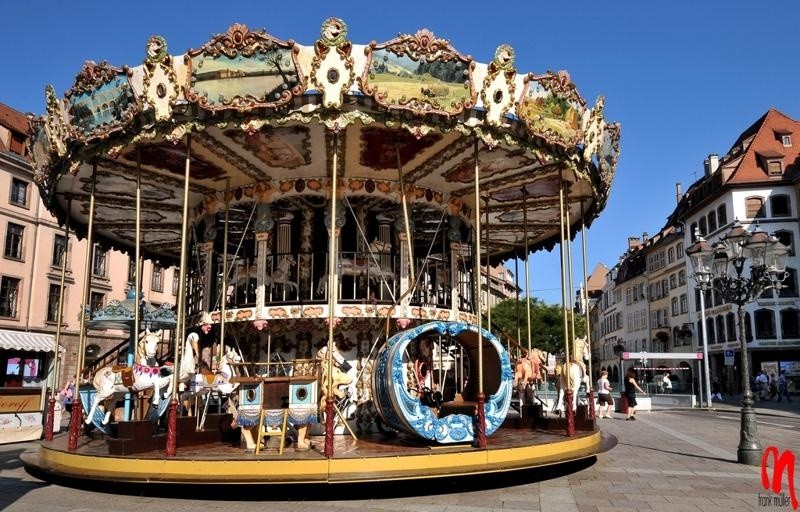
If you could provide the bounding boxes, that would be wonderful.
[681,214,795,468]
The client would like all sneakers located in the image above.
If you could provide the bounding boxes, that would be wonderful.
[626,416,636,421]
[599,414,614,420]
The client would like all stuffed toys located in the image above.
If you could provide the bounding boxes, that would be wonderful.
[81,326,592,451]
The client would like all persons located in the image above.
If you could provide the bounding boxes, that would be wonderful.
[662,371,673,393]
[710,376,727,402]
[624,366,647,420]
[750,369,794,402]
[81,326,592,451]
[62,373,77,432]
[656,381,663,394]
[597,371,614,419]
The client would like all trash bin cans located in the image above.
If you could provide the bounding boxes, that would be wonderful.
[620,390,629,414]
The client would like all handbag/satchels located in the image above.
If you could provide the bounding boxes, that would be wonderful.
[663,381,668,386]
